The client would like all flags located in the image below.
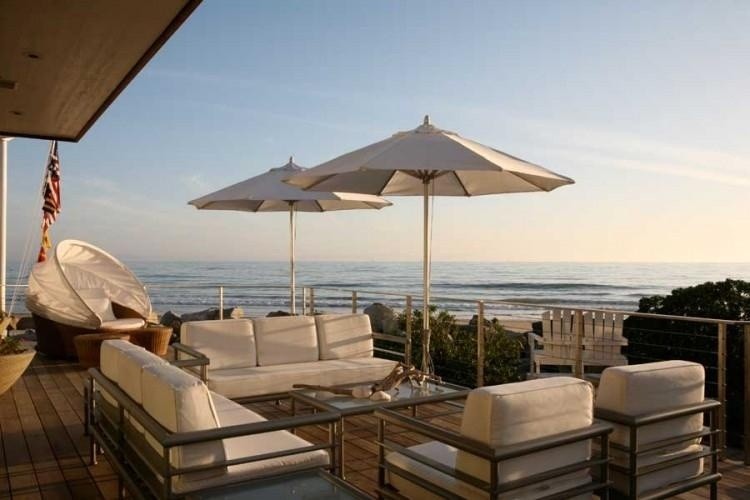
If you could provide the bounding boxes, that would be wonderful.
[37,140,64,263]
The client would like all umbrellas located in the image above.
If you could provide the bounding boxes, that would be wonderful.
[277,114,576,331]
[187,157,394,314]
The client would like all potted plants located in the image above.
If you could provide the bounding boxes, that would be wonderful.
[0,311,37,396]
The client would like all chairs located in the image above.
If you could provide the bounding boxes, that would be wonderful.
[25,239,152,361]
